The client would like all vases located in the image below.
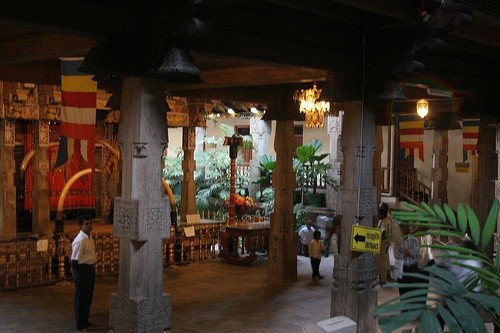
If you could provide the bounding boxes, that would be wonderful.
[236,210,244,222]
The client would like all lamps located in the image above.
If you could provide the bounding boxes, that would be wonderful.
[293,80,325,129]
[417,99,429,119]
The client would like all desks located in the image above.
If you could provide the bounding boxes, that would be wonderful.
[225,222,271,265]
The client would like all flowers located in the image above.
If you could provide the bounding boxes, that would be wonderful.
[223,194,257,216]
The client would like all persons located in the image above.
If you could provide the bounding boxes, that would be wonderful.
[299,219,315,257]
[71,214,96,331]
[377,202,483,323]
[308,232,325,280]
